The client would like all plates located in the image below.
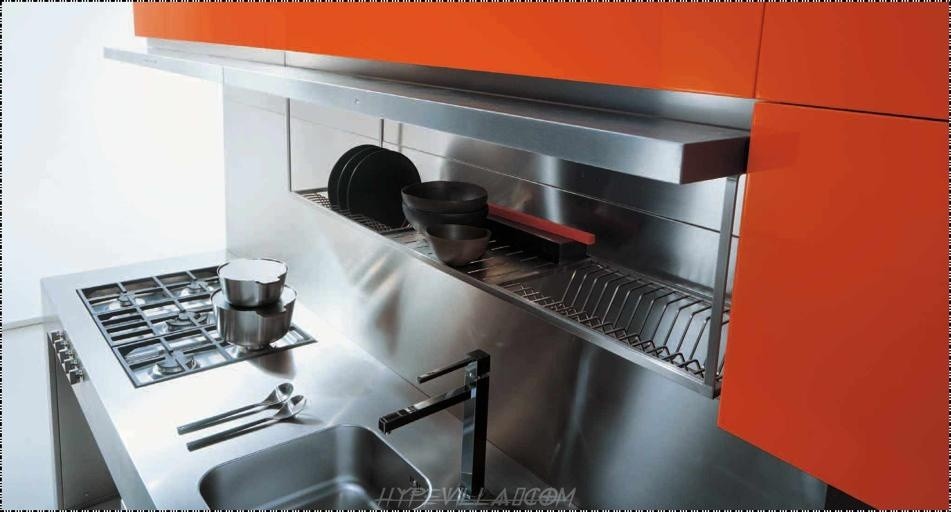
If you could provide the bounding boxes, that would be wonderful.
[328,143,372,206]
[338,147,387,211]
[348,150,422,229]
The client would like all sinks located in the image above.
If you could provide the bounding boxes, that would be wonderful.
[199,423,432,510]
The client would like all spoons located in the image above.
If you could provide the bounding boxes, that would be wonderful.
[187,395,307,452]
[177,383,293,434]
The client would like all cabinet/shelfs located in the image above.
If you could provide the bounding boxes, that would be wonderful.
[714,3,951,511]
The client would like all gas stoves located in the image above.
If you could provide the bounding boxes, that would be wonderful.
[72,259,320,390]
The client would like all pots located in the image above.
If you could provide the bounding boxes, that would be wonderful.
[211,253,302,354]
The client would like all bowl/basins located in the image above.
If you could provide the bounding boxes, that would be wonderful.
[400,181,489,211]
[426,224,492,264]
[401,204,490,234]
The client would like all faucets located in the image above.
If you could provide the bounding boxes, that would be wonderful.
[378,350,490,499]
